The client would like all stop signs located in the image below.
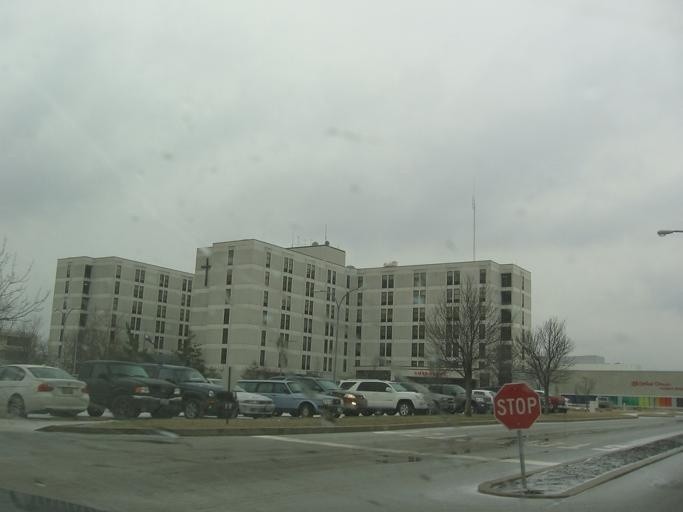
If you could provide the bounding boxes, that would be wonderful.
[494,383,541,431]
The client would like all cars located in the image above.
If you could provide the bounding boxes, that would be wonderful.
[472,389,497,406]
[535,390,570,414]
[0,363,90,419]
[206,378,275,418]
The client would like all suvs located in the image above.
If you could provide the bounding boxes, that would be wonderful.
[396,382,457,416]
[236,379,344,418]
[271,375,368,418]
[429,384,487,415]
[141,363,241,420]
[337,378,432,417]
[76,359,184,420]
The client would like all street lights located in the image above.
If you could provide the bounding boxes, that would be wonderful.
[656,229,683,239]
[312,286,366,381]
[51,306,85,364]
